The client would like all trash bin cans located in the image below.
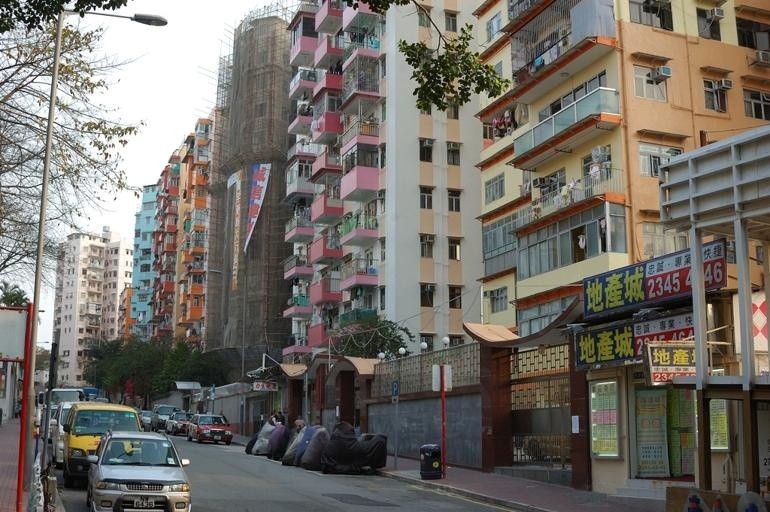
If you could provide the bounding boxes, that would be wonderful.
[419,444,442,480]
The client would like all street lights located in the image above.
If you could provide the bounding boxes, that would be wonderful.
[24,5,167,490]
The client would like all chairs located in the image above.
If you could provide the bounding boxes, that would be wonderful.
[110,442,159,464]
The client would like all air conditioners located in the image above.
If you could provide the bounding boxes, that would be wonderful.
[423,140,433,148]
[706,8,724,22]
[481,288,498,299]
[756,51,769,66]
[655,66,672,82]
[718,79,732,91]
[532,177,545,187]
[448,143,460,151]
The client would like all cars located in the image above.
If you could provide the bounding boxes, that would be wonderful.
[35,387,194,512]
[185,414,233,445]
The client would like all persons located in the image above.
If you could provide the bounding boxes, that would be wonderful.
[270,410,285,426]
[293,415,305,434]
